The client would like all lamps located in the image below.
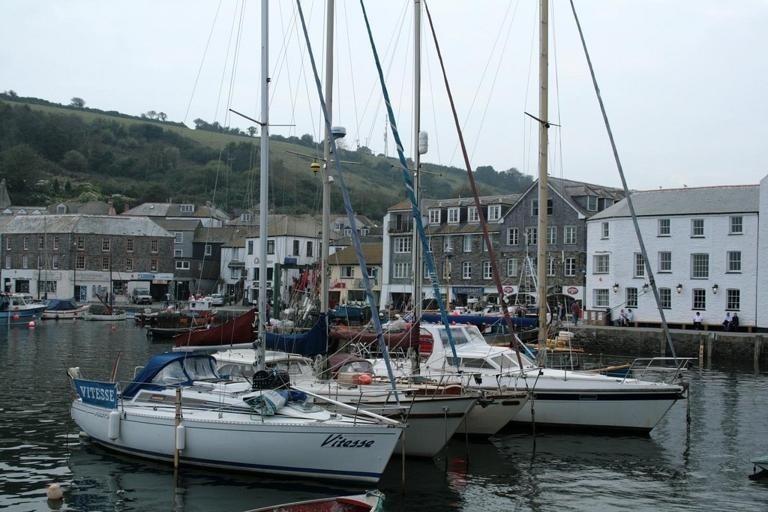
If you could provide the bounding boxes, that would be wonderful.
[611,281,620,293]
[710,283,720,294]
[640,282,650,293]
[676,282,683,295]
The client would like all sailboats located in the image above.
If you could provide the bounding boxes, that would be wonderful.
[77,253,132,322]
[160,0,700,511]
[64,2,407,483]
[41,261,92,320]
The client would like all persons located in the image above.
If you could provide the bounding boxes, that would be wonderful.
[722,312,732,330]
[619,308,625,326]
[187,292,209,308]
[623,309,633,327]
[693,312,704,331]
[554,299,583,327]
[265,301,273,325]
[728,312,739,331]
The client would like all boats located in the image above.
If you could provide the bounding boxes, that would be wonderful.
[1,292,47,323]
[248,489,385,510]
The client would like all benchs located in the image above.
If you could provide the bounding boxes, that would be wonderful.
[613,319,755,332]
